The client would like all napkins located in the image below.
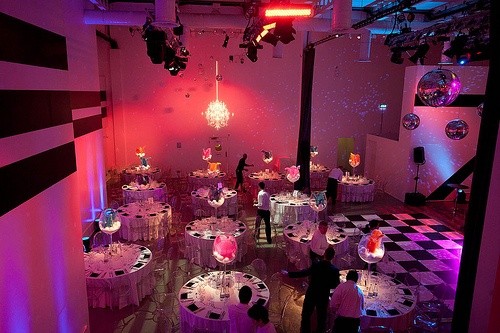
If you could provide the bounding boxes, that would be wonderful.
[185,281,198,288]
[397,287,413,295]
[222,270,232,275]
[253,283,267,290]
[242,274,253,282]
[388,278,402,285]
[209,309,222,320]
[382,305,400,316]
[90,272,101,278]
[253,296,266,305]
[371,271,380,277]
[200,271,210,278]
[114,269,124,276]
[142,253,151,258]
[187,302,201,312]
[395,297,414,307]
[180,291,194,300]
[133,262,144,268]
[138,246,147,251]
[366,310,378,317]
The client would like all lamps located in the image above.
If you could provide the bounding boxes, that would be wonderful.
[142,6,491,165]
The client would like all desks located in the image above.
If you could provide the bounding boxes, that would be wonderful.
[336,173,376,204]
[183,215,247,268]
[282,218,350,270]
[309,163,332,190]
[176,269,271,333]
[120,165,163,186]
[190,184,239,218]
[116,199,173,242]
[327,268,418,333]
[186,169,229,191]
[121,180,169,205]
[268,191,319,226]
[82,243,156,309]
[246,168,287,201]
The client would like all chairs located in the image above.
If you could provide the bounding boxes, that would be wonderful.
[83,165,450,333]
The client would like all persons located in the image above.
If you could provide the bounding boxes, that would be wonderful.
[310,220,328,265]
[329,271,364,333]
[283,247,340,333]
[247,304,276,333]
[234,154,253,191]
[326,166,344,208]
[228,285,257,333]
[362,219,379,271]
[253,182,272,244]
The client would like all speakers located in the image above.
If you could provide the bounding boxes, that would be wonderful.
[414,147,425,163]
